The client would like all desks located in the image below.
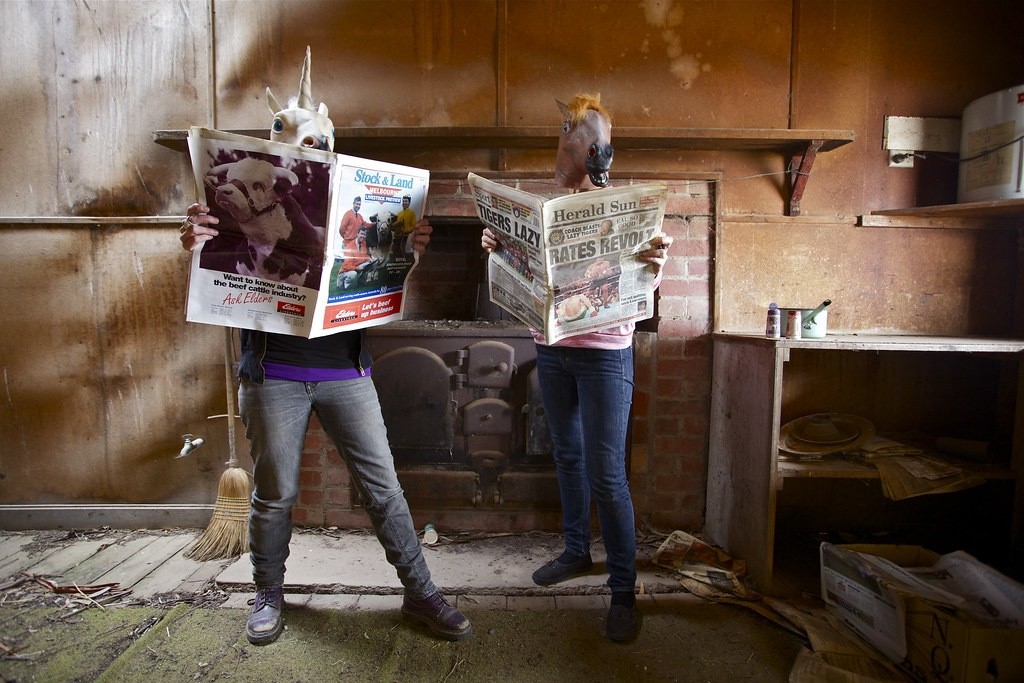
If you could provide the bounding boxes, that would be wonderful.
[704,331,1024,599]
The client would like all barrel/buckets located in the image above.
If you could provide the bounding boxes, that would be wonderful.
[955,84,1024,201]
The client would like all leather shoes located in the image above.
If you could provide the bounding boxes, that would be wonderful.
[245,586,285,645]
[401,591,472,640]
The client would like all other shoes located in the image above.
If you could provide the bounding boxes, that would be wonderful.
[607,595,639,643]
[604,303,610,308]
[531,554,594,585]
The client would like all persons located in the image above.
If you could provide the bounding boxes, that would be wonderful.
[485,223,533,282]
[556,257,612,323]
[178,101,473,646]
[480,96,674,645]
[337,226,372,292]
[601,219,615,236]
[395,194,417,268]
[339,196,377,252]
[638,300,647,311]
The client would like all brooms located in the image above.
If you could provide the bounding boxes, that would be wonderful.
[183,326,255,562]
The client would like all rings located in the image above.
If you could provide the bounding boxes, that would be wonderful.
[181,216,198,230]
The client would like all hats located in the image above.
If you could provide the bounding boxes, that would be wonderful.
[792,415,859,443]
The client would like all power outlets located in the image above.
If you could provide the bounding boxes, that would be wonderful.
[889,150,914,168]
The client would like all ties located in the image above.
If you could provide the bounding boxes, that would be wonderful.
[355,211,357,218]
[359,243,361,251]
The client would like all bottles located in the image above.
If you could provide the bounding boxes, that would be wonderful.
[785,310,801,340]
[766,303,780,338]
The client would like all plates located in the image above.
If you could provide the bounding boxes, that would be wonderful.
[778,411,873,457]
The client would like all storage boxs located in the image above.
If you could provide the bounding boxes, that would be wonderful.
[957,83,1024,203]
[820,541,1024,683]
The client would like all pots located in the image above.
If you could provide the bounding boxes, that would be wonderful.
[777,297,832,338]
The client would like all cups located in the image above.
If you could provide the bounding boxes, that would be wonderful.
[424,524,438,544]
